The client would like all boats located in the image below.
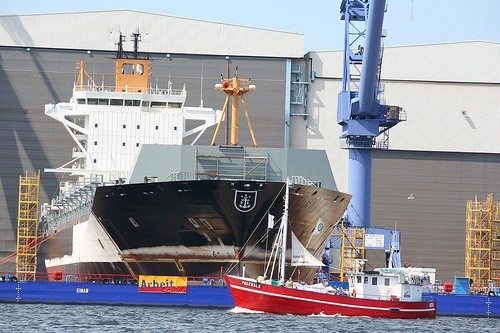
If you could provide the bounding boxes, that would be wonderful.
[223,177,436,319]
[27,27,351,283]
